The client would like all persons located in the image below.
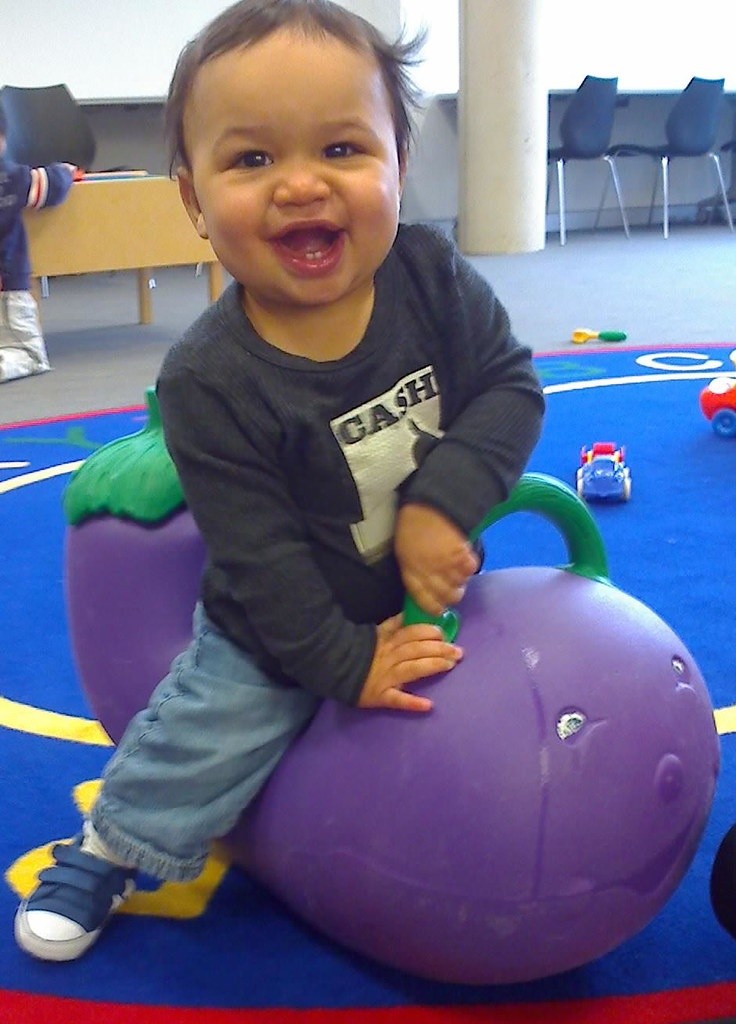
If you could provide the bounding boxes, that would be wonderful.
[0,101,81,384]
[10,0,547,964]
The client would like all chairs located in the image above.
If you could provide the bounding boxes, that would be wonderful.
[0,82,136,298]
[547,75,631,246]
[592,76,736,239]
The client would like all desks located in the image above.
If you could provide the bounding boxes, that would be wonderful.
[21,175,225,326]
[436,87,736,101]
[75,93,167,109]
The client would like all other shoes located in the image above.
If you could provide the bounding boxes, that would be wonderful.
[14,831,136,961]
